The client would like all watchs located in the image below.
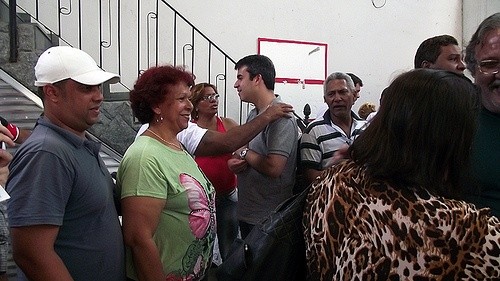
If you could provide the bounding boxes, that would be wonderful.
[239,147,249,160]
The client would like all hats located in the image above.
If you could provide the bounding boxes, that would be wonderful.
[34,46,121,87]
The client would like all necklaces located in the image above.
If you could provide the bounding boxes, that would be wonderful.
[147,129,187,153]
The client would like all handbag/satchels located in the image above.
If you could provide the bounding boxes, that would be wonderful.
[216,190,306,281]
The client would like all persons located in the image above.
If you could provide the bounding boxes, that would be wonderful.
[0,115,33,281]
[347,73,363,121]
[135,103,294,156]
[189,83,245,262]
[5,46,127,281]
[302,69,500,281]
[464,12,500,86]
[226,55,298,240]
[117,65,222,281]
[415,35,466,74]
[299,72,372,185]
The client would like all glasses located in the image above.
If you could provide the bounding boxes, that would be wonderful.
[474,60,500,75]
[201,94,219,100]
